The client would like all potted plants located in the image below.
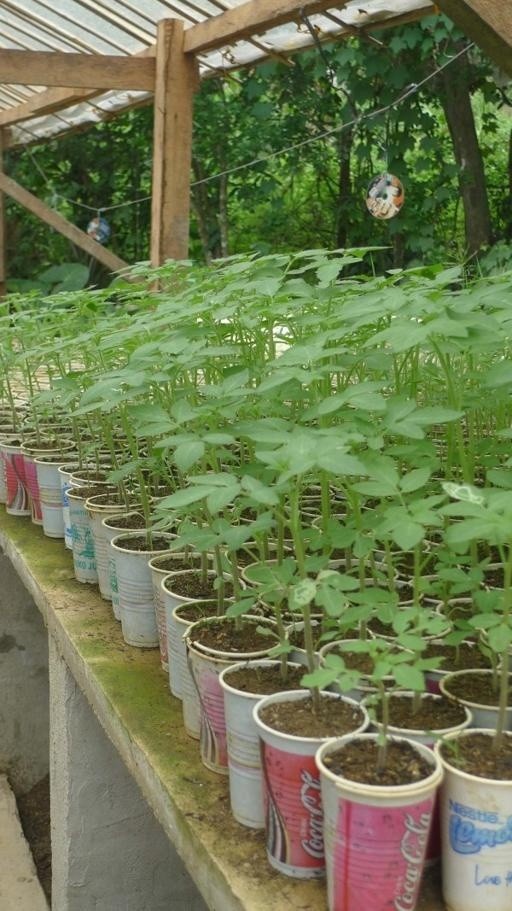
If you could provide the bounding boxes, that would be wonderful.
[0,240,512,911]
